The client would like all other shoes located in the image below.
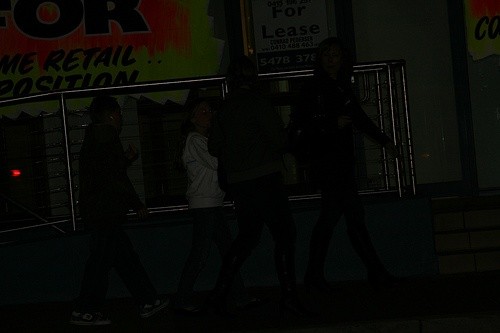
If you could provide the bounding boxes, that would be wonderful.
[139,298,170,319]
[70,310,113,326]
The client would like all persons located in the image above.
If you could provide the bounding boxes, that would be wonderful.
[172,38,407,321]
[70,95,170,327]
